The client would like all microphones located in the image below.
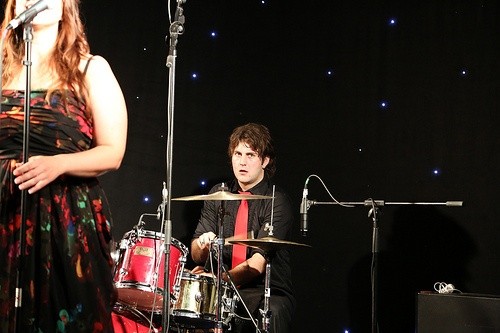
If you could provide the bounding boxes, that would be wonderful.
[5,0,54,32]
[300,186,309,238]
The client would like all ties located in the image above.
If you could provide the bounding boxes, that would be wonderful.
[231,191,254,289]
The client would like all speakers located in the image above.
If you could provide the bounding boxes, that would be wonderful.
[416,292,500,333]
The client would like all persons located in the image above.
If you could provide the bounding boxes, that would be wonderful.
[0,0,127,333]
[190,122,296,333]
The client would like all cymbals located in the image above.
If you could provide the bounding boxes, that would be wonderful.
[228,238,313,247]
[169,191,276,201]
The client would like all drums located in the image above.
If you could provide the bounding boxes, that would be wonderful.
[92,300,158,333]
[114,228,190,309]
[108,239,122,272]
[173,271,235,327]
